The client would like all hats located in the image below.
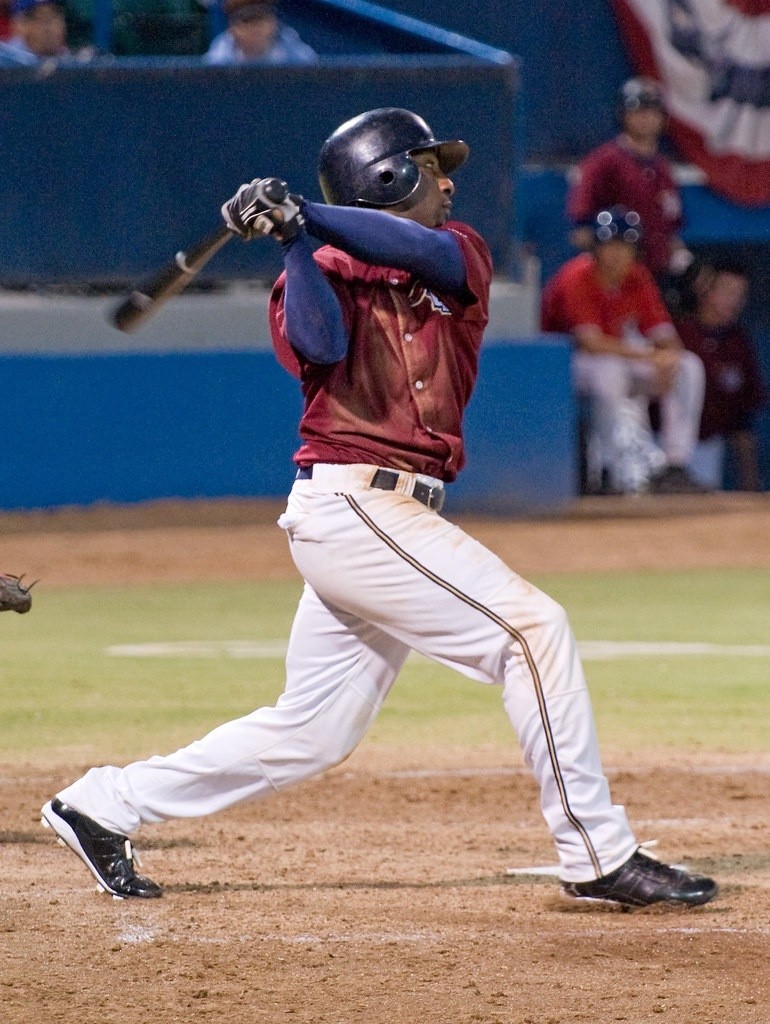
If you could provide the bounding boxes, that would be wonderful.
[318,107,469,207]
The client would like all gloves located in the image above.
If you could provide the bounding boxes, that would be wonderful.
[220,176,306,242]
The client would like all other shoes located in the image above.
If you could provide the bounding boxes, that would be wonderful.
[646,465,709,496]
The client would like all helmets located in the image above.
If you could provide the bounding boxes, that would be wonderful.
[594,204,641,246]
[619,76,665,110]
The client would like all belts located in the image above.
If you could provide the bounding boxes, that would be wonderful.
[295,465,446,512]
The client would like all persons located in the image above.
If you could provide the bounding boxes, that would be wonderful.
[0,0,319,83]
[542,76,765,498]
[37,108,717,910]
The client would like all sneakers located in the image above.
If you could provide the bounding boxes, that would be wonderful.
[559,846,718,909]
[41,794,163,902]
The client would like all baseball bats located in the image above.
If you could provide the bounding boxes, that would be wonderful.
[103,179,288,332]
